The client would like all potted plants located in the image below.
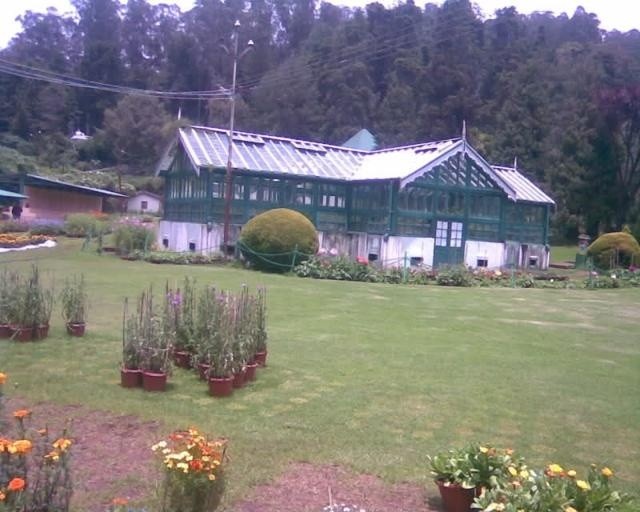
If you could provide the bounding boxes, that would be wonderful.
[1,262,87,342]
[426,448,484,512]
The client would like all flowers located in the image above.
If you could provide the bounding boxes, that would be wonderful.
[121,275,266,374]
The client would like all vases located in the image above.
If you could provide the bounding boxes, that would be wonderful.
[112,343,267,397]
